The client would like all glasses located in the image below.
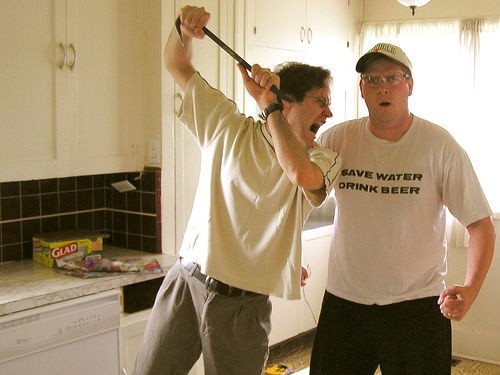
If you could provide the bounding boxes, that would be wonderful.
[296,94,331,108]
[359,70,409,88]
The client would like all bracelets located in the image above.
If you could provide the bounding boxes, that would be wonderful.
[257,102,283,121]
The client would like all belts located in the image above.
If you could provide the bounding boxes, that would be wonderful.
[180,256,261,297]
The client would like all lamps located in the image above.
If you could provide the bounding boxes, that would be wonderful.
[397,0,431,16]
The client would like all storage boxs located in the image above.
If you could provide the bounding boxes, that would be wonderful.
[33,230,102,268]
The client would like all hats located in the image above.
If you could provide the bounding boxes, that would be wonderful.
[355,43,412,76]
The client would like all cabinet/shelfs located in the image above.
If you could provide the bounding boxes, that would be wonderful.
[0,0,359,375]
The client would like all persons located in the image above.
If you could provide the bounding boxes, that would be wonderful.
[132,5,346,375]
[301,43,496,375]
